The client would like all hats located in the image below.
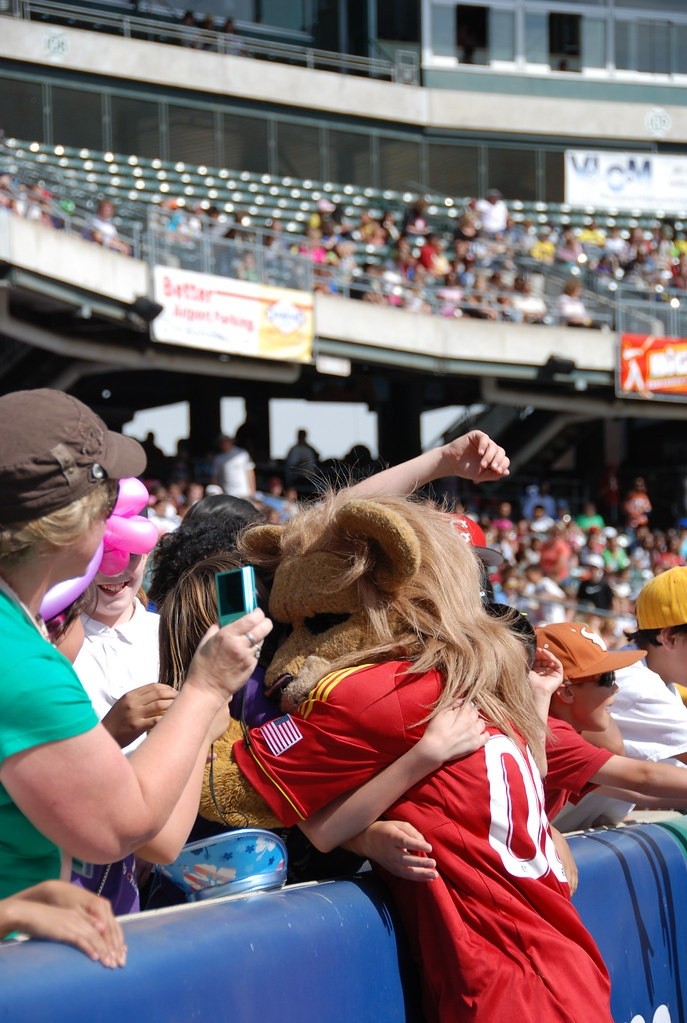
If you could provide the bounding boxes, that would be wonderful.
[534,620,649,682]
[450,512,504,568]
[636,563,687,631]
[585,554,602,571]
[154,827,289,904]
[0,389,146,525]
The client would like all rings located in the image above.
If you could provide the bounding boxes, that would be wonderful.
[256,646,261,650]
[244,632,256,645]
[255,651,259,658]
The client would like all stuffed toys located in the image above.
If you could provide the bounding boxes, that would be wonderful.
[148,447,612,1023]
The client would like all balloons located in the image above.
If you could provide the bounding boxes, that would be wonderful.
[38,476,159,622]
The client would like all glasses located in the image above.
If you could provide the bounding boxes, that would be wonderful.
[569,669,615,688]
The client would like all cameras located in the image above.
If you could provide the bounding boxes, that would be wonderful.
[215,566,258,628]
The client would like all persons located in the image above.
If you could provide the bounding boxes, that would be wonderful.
[480,605,687,832]
[450,241,547,324]
[175,9,244,53]
[457,187,512,240]
[608,565,687,766]
[0,173,452,313]
[520,219,687,327]
[141,430,612,883]
[1,388,270,971]
[449,475,687,647]
[141,420,374,523]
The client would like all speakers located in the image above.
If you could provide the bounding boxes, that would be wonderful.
[547,357,576,375]
[131,296,164,322]
